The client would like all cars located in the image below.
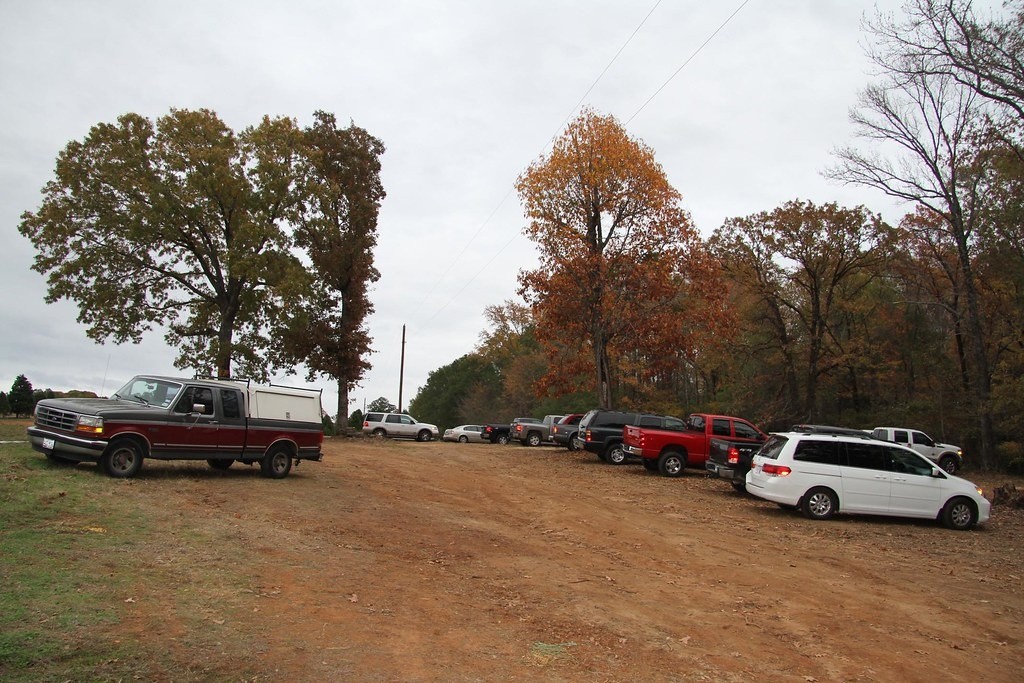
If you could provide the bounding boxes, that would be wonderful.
[744,431,991,530]
[442,424,491,444]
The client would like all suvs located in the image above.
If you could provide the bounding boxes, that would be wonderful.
[362,412,439,442]
[577,408,688,465]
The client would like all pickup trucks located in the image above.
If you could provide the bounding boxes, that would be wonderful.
[862,426,966,475]
[704,425,902,493]
[620,412,769,477]
[26,372,326,480]
[480,412,585,452]
